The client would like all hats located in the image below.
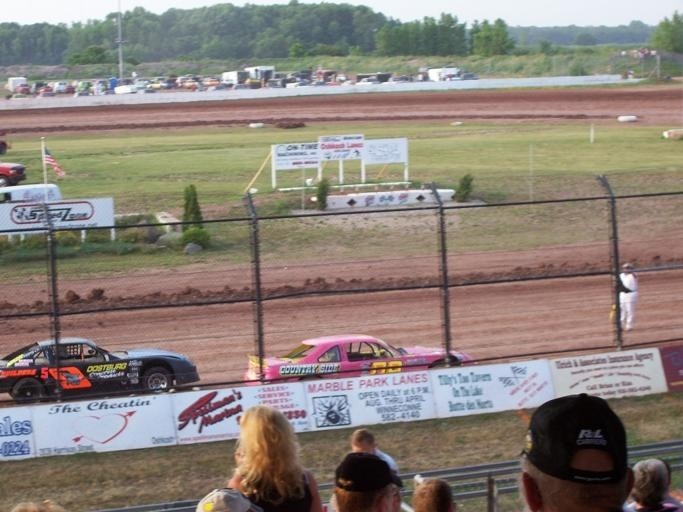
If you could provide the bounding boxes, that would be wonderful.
[195,488,263,512]
[521,394,627,484]
[336,453,403,491]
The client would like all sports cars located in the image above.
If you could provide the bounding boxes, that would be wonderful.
[245,334,474,386]
[0,338,199,403]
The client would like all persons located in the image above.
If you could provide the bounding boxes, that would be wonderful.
[516,392,635,512]
[193,404,403,512]
[622,458,683,512]
[618,263,638,333]
[6,499,69,512]
[411,478,457,512]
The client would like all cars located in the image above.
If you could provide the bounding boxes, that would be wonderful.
[0,164,26,184]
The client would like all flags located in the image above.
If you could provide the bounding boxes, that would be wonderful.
[45,146,67,178]
